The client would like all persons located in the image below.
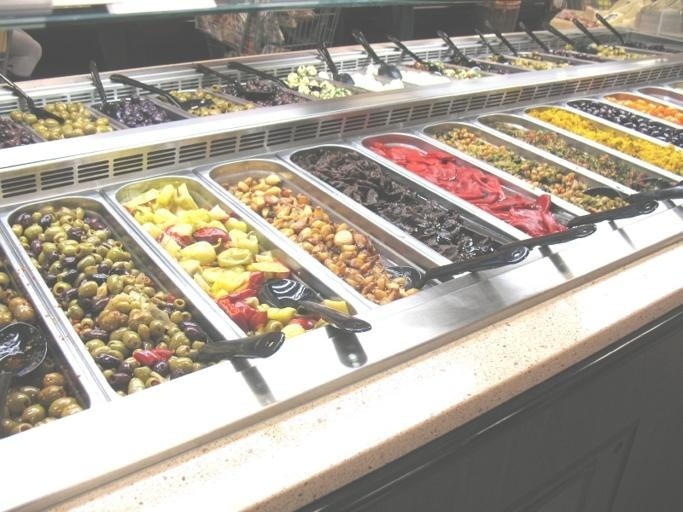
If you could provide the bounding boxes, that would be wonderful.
[6,30,42,80]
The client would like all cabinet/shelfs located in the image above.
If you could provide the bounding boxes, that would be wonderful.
[0,2,683,512]
